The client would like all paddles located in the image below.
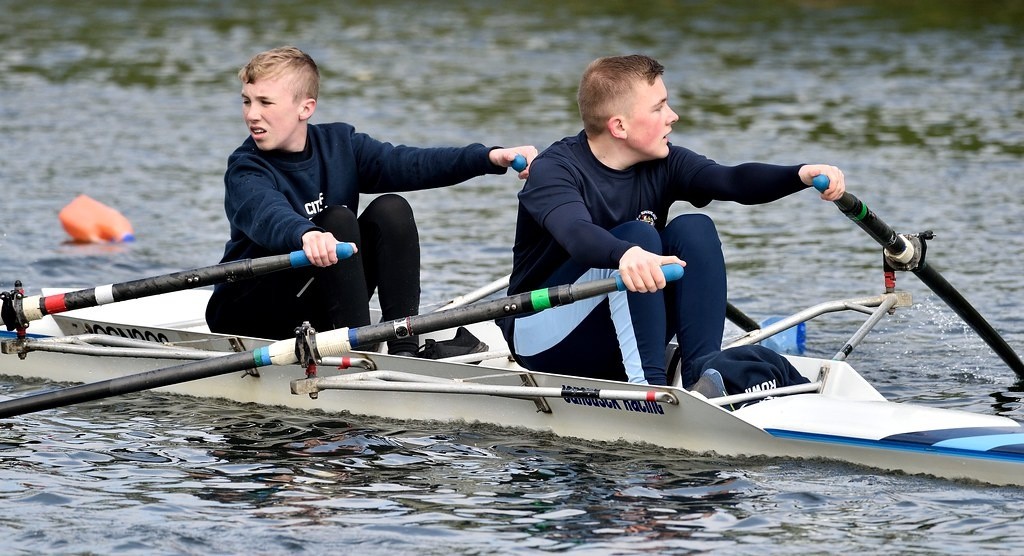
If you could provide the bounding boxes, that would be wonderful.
[0,258,687,420]
[0,240,357,335]
[809,172,1024,380]
[512,151,810,359]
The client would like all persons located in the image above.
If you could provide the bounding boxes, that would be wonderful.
[503,54,846,395]
[206,45,537,358]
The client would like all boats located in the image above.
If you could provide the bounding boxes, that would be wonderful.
[1,281,1024,496]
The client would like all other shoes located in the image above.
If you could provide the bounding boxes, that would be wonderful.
[416,326,489,366]
[693,367,726,407]
